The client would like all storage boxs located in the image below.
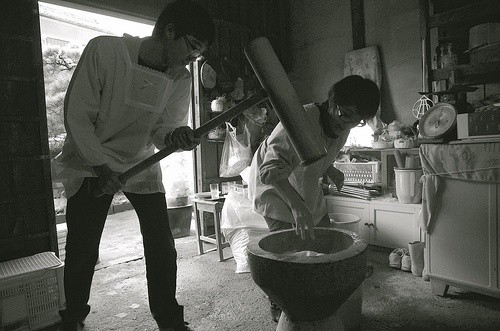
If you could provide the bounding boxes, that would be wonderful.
[0,251,67,330]
[334,159,382,183]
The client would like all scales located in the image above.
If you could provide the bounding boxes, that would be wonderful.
[418,86,478,144]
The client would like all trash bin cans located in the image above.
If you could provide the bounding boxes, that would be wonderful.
[327,212,361,234]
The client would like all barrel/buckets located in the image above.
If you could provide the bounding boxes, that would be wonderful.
[328,212,362,235]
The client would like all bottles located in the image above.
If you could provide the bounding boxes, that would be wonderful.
[439,42,453,68]
[211,100,223,112]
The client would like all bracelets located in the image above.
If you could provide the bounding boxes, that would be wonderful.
[169,128,176,143]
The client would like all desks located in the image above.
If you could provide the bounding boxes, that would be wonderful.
[192,196,231,261]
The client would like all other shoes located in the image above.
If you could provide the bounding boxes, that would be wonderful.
[159,321,190,331]
[58,320,84,331]
[401,251,411,271]
[388,247,404,269]
[269,302,284,322]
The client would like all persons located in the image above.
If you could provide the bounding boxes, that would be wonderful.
[56,0,215,331]
[248,74,381,323]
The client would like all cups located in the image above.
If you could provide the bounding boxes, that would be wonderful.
[393,168,423,204]
[209,183,219,199]
[222,181,236,195]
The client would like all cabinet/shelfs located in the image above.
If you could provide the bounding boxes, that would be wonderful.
[325,194,424,248]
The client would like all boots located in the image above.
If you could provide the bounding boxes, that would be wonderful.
[409,241,424,276]
[422,247,429,281]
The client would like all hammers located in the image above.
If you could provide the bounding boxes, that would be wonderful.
[91,35,329,199]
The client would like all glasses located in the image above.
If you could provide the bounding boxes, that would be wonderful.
[336,105,366,127]
[182,33,204,61]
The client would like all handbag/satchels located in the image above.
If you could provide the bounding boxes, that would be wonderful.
[219,121,253,178]
